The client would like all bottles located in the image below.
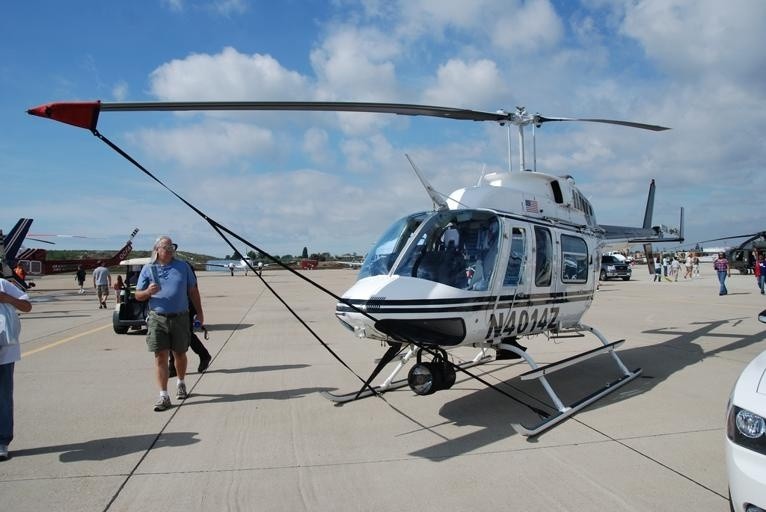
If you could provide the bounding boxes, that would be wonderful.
[192,313,201,333]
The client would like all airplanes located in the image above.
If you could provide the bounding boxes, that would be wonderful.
[191,244,296,276]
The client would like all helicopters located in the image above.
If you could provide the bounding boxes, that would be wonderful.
[26,100,684,437]
[672,231,766,274]
[0,218,139,293]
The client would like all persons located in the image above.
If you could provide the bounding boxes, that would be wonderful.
[74,265,86,295]
[0,277,33,461]
[169,243,212,376]
[114,275,124,303]
[14,263,31,281]
[92,260,111,309]
[714,251,730,295]
[653,254,701,283]
[755,255,766,293]
[135,235,204,411]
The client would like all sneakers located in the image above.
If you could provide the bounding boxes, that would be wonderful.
[154,396,170,410]
[198,355,211,371]
[176,384,186,399]
[99,301,106,309]
[0,445,8,457]
[168,370,176,377]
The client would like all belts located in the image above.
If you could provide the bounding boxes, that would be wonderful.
[154,312,186,318]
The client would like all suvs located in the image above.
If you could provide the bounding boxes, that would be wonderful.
[600,255,632,281]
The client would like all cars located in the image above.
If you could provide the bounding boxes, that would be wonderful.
[725,309,766,512]
